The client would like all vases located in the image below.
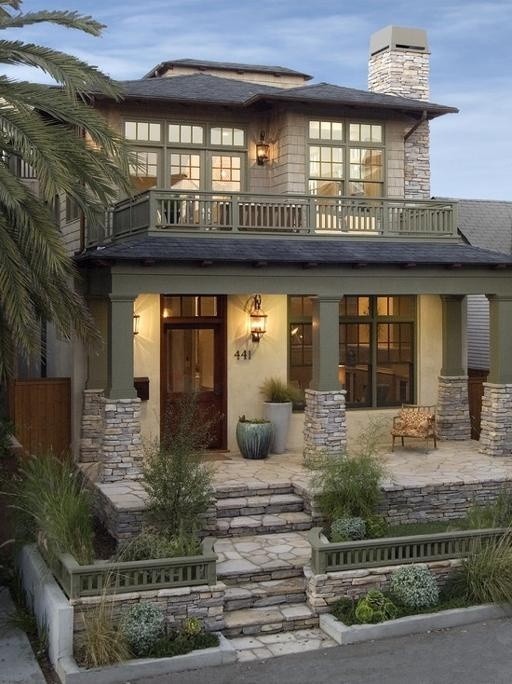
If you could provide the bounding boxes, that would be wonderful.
[236,420,272,460]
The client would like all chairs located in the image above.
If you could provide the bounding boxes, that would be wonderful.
[391,404,437,452]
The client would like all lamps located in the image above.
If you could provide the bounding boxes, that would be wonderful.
[244,295,267,343]
[256,131,270,165]
[134,315,140,335]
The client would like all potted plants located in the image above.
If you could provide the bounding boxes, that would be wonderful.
[259,377,293,454]
